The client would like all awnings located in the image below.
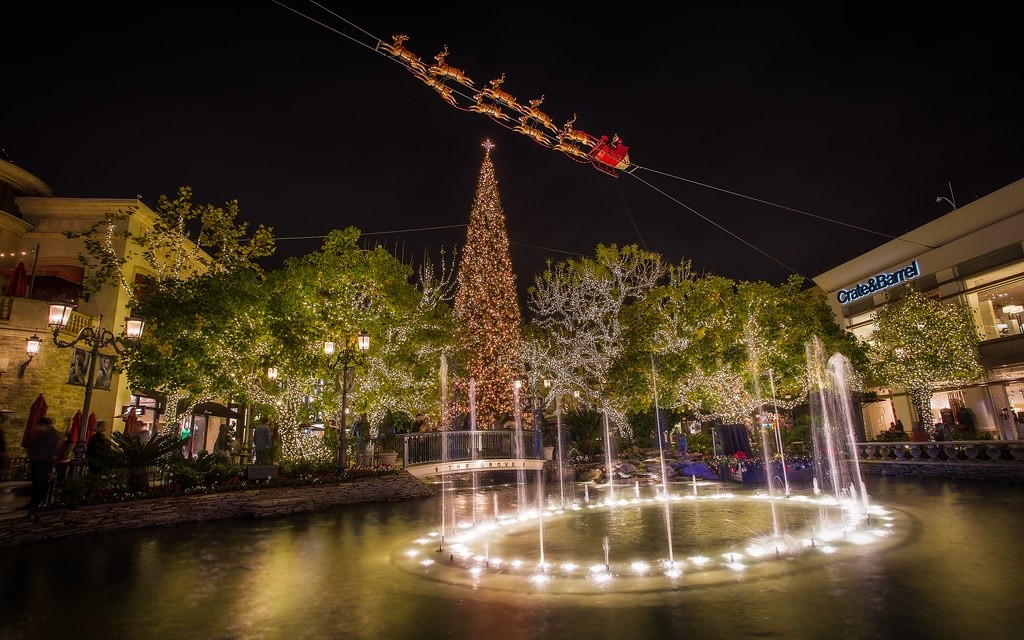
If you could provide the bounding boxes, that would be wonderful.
[0,266,83,288]
[133,387,244,420]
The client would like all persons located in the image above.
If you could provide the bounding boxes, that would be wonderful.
[957,407,976,434]
[611,133,619,149]
[249,415,281,483]
[933,423,945,441]
[410,413,433,464]
[84,421,109,473]
[350,412,370,467]
[20,417,63,511]
[891,419,903,431]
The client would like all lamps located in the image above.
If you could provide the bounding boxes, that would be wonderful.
[16,333,43,379]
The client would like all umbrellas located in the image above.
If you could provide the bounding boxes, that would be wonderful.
[4,259,26,298]
[67,409,97,447]
[21,392,49,449]
[122,404,139,435]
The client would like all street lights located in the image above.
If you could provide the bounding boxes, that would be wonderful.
[47,291,145,486]
[324,328,370,469]
[229,362,279,471]
[516,375,552,432]
